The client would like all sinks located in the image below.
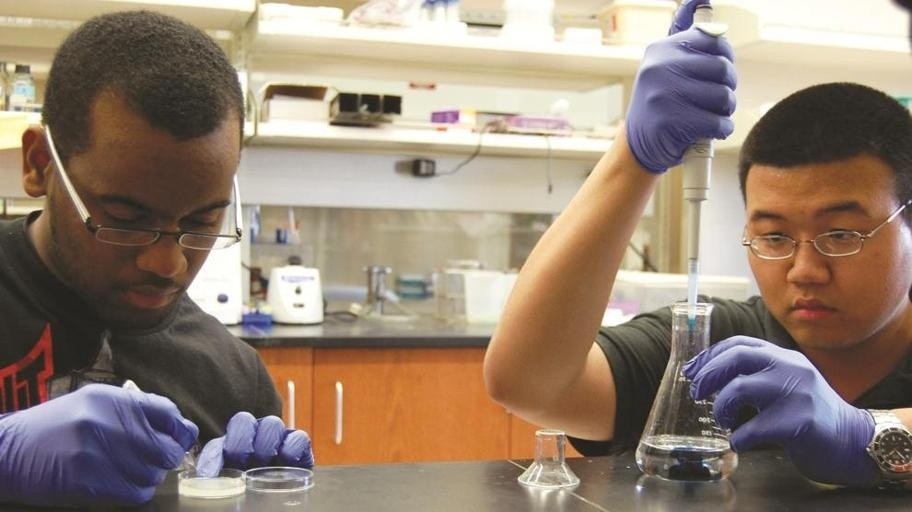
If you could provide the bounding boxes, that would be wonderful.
[377,311,419,326]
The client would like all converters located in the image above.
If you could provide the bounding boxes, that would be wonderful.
[413,159,435,176]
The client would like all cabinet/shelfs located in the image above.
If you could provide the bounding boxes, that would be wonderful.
[242,347,511,465]
[0,0,257,150]
[241,0,678,160]
[513,414,582,460]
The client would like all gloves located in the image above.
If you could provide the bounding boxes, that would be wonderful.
[681,335,877,485]
[626,0,736,173]
[0,382,200,504]
[196,413,315,477]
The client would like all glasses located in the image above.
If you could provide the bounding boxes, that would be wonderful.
[742,196,912,256]
[43,123,243,250]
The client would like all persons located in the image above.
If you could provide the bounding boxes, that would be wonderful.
[1,11,313,501]
[484,0,912,489]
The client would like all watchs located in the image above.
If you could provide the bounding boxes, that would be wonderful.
[867,404,912,490]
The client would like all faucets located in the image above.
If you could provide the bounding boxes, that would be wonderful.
[362,262,401,316]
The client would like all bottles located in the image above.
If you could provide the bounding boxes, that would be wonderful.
[0,61,36,112]
[635,302,739,483]
